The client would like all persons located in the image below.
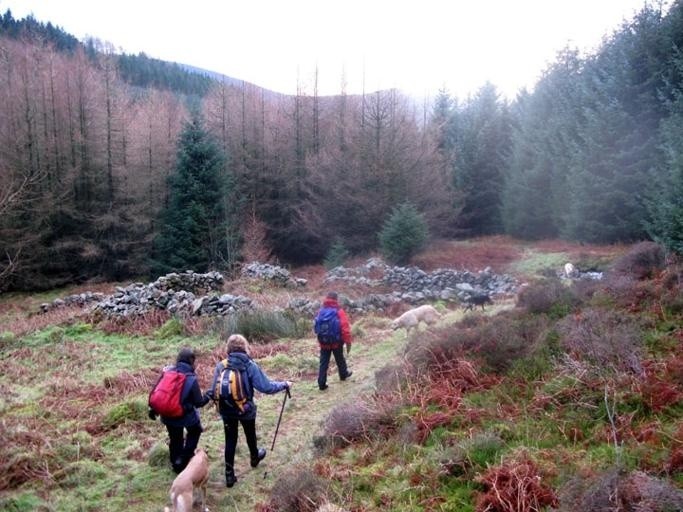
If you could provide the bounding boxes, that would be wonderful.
[315,290,352,390]
[210,333,292,488]
[147,348,214,476]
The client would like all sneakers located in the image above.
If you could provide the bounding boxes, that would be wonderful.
[250,447,266,467]
[339,371,352,380]
[226,476,237,487]
[318,384,328,390]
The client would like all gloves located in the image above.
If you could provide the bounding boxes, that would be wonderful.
[149,408,159,420]
[206,389,215,401]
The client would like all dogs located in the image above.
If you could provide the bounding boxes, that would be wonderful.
[565,263,580,278]
[164,446,210,512]
[391,305,442,338]
[462,290,494,313]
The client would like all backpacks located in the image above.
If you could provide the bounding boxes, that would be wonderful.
[314,306,342,344]
[147,367,194,419]
[213,358,254,418]
